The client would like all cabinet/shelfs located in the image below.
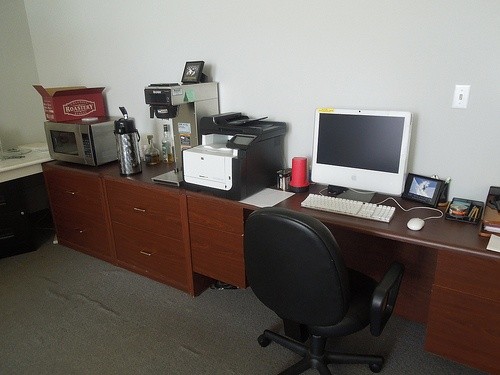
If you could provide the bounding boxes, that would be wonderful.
[42,158,500,375]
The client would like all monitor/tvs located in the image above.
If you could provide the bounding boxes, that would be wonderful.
[310,110,413,203]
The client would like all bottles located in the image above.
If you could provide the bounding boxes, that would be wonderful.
[161,124,174,163]
[144,135,159,165]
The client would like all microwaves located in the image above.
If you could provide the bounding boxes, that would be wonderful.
[44,116,118,166]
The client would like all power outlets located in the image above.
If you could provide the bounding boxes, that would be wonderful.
[453,85,471,109]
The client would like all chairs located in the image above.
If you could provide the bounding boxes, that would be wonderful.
[244,207,405,375]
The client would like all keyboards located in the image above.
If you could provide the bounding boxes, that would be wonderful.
[301,194,396,223]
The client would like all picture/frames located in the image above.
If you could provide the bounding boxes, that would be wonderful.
[181,61,208,84]
[401,173,445,207]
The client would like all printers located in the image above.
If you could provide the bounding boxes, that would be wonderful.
[180,113,287,200]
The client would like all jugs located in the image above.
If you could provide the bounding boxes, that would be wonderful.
[114,107,143,176]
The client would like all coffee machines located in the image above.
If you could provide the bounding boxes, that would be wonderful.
[143,82,221,188]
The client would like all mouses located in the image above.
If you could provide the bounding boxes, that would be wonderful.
[406,217,425,231]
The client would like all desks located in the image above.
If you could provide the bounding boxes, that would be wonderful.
[0,142,58,244]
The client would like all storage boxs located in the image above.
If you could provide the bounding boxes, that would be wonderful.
[32,85,105,122]
